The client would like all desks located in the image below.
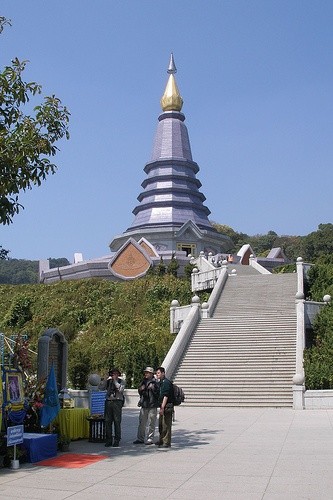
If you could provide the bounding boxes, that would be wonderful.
[2,433,58,464]
[57,409,90,440]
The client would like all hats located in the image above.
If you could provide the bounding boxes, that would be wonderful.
[144,367,154,374]
[109,368,121,376]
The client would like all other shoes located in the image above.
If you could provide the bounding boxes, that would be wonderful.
[113,441,119,446]
[161,444,170,448]
[133,440,144,444]
[155,440,163,445]
[105,442,112,447]
[145,440,153,445]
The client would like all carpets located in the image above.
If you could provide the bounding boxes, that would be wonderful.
[31,452,108,469]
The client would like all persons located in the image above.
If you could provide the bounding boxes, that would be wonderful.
[98,368,125,447]
[226,254,234,264]
[155,367,175,448]
[133,367,159,445]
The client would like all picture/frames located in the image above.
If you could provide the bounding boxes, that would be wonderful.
[6,372,24,406]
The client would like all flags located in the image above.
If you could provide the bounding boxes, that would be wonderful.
[39,367,60,428]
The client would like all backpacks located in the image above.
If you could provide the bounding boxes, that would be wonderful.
[171,382,185,406]
